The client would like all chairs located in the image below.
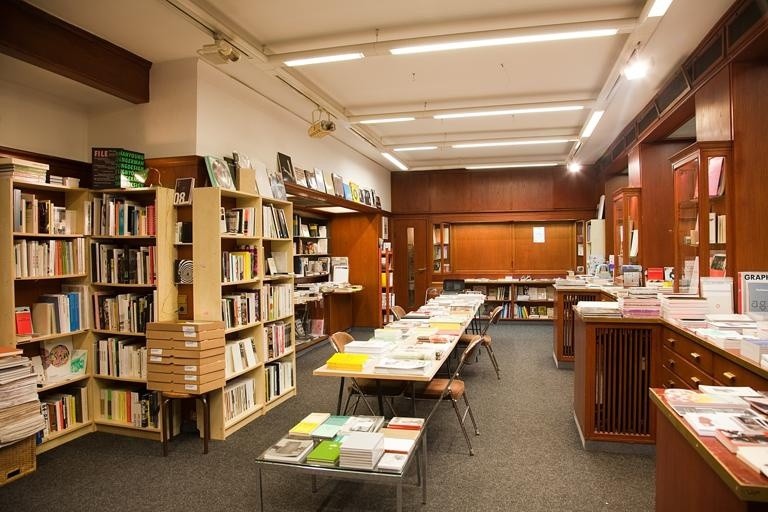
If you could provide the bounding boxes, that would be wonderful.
[457,306,503,381]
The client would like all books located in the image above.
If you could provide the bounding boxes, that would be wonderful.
[479,273,576,320]
[262,412,425,472]
[380,242,395,323]
[0,148,159,446]
[293,214,349,338]
[559,274,768,369]
[277,152,381,210]
[665,386,768,477]
[327,294,480,375]
[205,151,294,421]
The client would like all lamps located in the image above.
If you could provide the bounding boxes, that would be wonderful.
[196,32,241,66]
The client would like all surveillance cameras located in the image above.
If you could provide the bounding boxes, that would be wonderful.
[308,120,336,138]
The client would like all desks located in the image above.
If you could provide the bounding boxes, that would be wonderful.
[255,413,427,511]
[314,294,488,486]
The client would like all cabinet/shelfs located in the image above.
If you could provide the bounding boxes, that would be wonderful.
[430,273,586,322]
[432,222,450,274]
